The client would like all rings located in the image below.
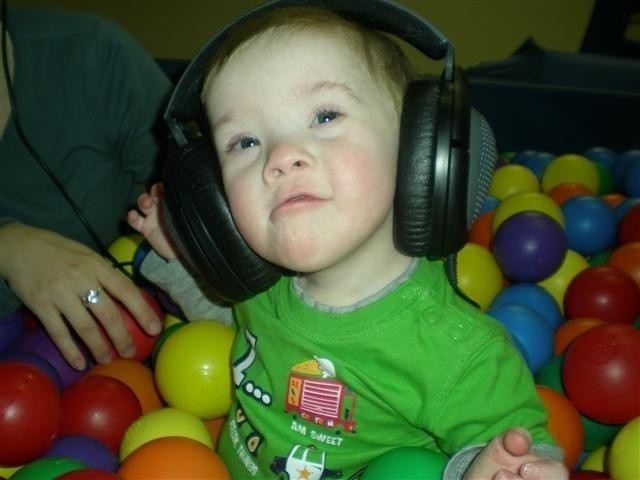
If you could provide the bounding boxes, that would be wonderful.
[83,288,102,306]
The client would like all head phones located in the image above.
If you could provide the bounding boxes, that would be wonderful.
[158,0,499,305]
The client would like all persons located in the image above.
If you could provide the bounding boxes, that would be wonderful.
[125,7,570,480]
[0,6,173,370]
